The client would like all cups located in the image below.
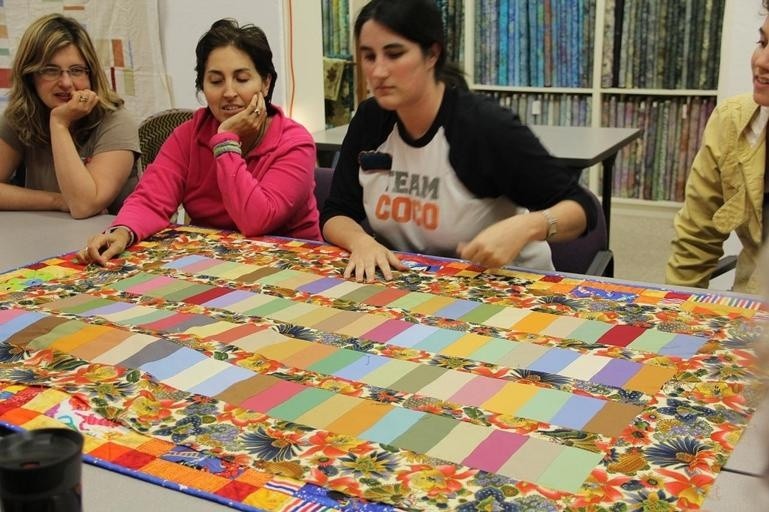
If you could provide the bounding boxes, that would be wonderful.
[0,427,84,512]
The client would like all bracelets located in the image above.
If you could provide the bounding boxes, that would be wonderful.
[104,225,135,249]
[541,209,561,241]
[211,138,244,159]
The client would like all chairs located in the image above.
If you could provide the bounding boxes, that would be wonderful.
[549,186,615,278]
[136,107,199,179]
[313,165,372,236]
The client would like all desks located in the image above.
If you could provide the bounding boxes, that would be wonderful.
[3,203,768,510]
[312,117,645,250]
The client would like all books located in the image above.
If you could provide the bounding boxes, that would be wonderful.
[601,1,725,202]
[321,1,466,129]
[474,2,596,128]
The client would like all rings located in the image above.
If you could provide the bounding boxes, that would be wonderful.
[254,108,261,116]
[78,96,89,102]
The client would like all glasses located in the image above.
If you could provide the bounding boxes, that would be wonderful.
[38,67,91,81]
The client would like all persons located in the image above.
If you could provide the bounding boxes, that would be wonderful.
[73,18,326,268]
[1,13,144,220]
[663,0,769,299]
[316,2,599,284]
